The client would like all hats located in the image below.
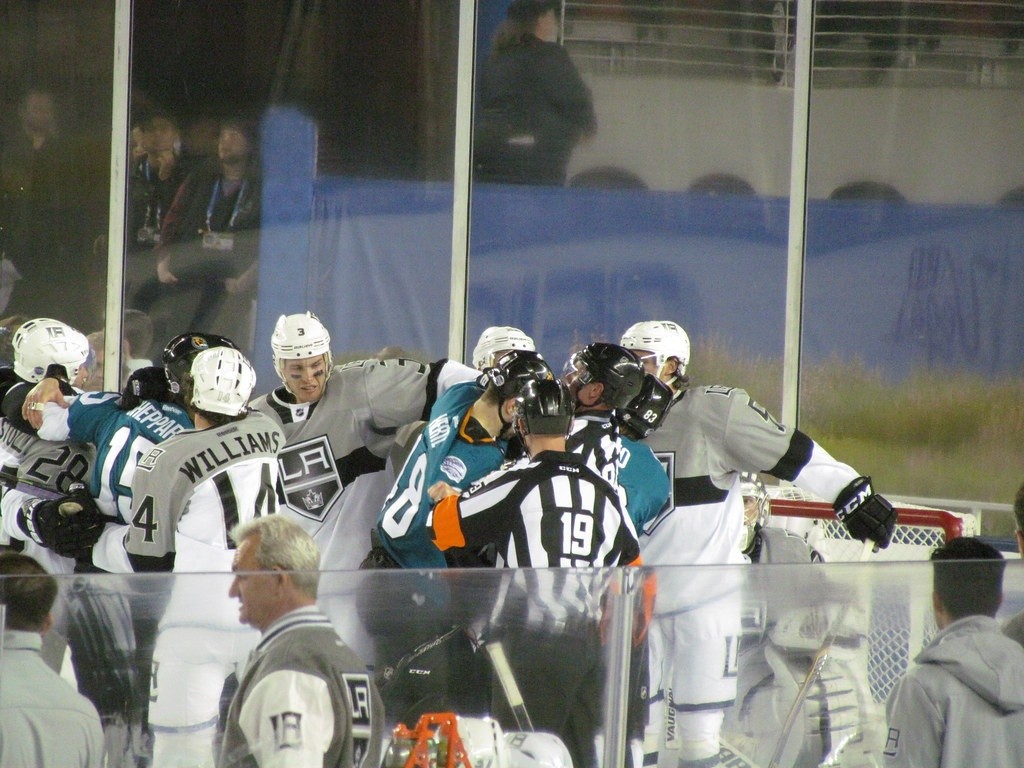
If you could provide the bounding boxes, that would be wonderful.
[127,368,172,403]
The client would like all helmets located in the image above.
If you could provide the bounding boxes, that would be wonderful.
[515,377,574,417]
[739,471,770,531]
[487,349,556,404]
[473,326,537,371]
[269,311,333,383]
[12,317,96,389]
[162,333,241,398]
[620,320,691,375]
[614,374,673,438]
[563,343,644,410]
[189,347,258,418]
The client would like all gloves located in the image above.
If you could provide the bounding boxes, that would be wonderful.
[26,494,104,562]
[832,478,896,549]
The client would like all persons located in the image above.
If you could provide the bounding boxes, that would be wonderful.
[1,309,903,767]
[473,2,596,186]
[1001,482,1024,648]
[0,87,267,294]
[882,536,1024,768]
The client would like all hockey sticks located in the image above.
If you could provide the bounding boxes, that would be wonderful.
[484,623,535,733]
[767,537,874,768]
